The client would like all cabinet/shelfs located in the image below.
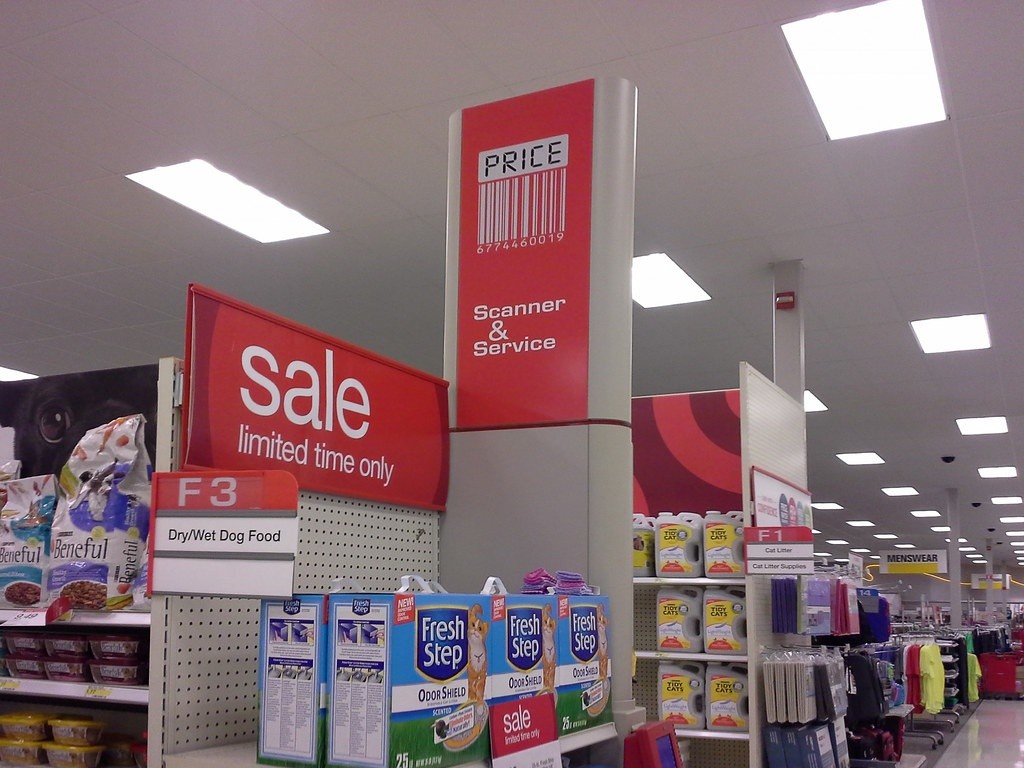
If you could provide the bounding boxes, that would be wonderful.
[631,358,859,768]
[939,640,966,724]
[0,281,622,768]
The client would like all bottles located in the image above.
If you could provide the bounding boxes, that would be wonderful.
[653,511,703,578]
[658,660,707,730]
[703,586,746,654]
[707,511,745,578]
[705,660,753,732]
[657,586,704,654]
[633,513,656,577]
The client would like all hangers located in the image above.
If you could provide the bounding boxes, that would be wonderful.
[865,633,936,647]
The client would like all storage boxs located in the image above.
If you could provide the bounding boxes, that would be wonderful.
[0,632,145,686]
[254,588,612,768]
[0,710,147,768]
